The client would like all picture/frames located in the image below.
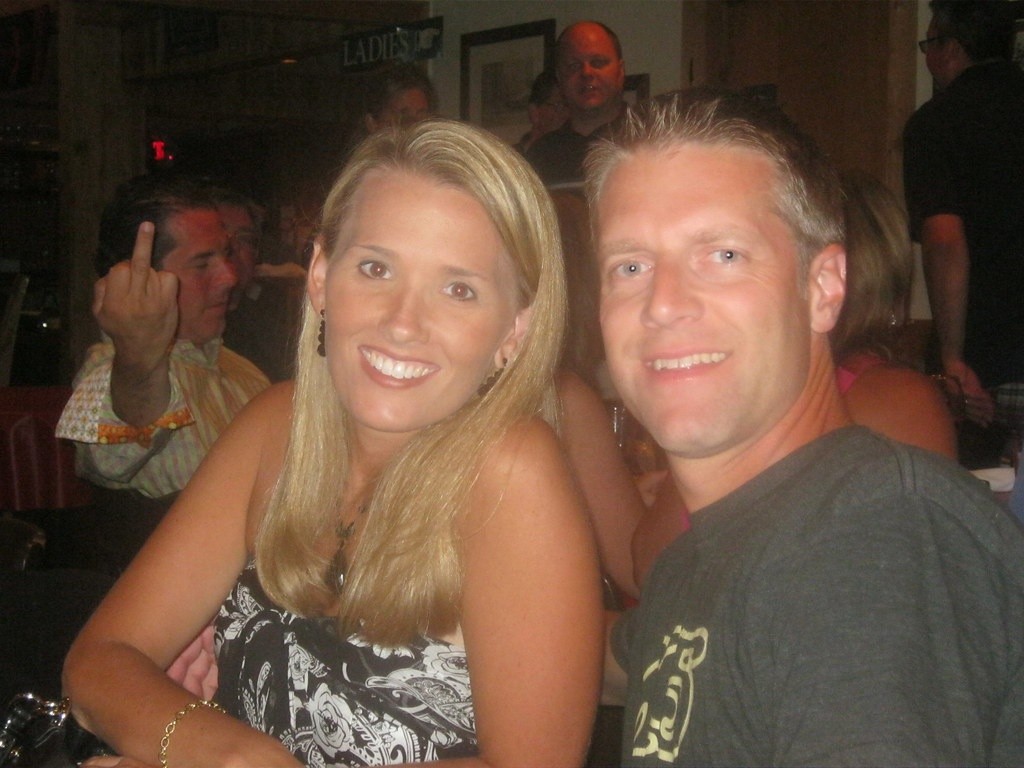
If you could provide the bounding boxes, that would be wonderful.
[459,19,557,149]
[621,71,650,117]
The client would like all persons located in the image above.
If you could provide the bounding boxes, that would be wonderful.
[55,185,273,498]
[276,59,434,270]
[54,1,1024,601]
[166,118,1024,768]
[512,17,636,179]
[60,116,605,768]
[896,2,1024,446]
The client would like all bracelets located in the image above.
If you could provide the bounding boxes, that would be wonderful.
[158,700,228,767]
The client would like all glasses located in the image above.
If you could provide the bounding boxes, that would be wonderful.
[545,95,568,112]
[918,35,948,54]
